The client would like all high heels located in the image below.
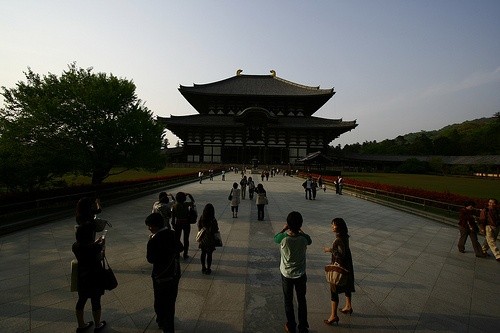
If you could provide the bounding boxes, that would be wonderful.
[324,317,339,325]
[339,308,353,317]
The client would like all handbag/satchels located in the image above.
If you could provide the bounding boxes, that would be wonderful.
[324,261,349,287]
[190,203,197,224]
[213,231,222,247]
[196,227,204,242]
[70,262,77,291]
[96,254,118,290]
[229,193,232,200]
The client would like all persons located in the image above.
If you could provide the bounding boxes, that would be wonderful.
[458,198,500,261]
[230,183,240,218]
[261,167,291,181]
[254,184,266,221]
[72,197,108,333]
[302,175,326,200]
[221,168,225,181]
[273,211,313,333]
[145,212,184,333]
[323,217,355,326]
[231,164,238,174]
[199,170,203,184]
[240,176,255,200]
[152,190,196,261]
[198,203,219,274]
[333,175,344,195]
[208,167,213,181]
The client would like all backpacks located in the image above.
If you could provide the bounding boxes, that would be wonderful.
[259,195,267,203]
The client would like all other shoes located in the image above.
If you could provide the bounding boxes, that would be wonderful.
[206,269,211,274]
[458,244,500,261]
[202,268,206,273]
[283,324,295,333]
[76,321,93,333]
[94,320,106,333]
